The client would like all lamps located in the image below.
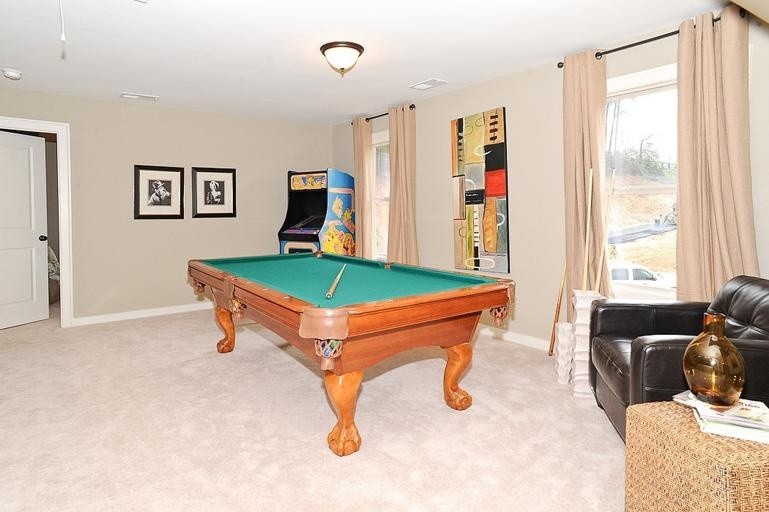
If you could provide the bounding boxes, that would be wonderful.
[321,40,364,75]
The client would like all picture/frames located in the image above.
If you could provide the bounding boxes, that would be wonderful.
[134,165,184,220]
[190,167,236,218]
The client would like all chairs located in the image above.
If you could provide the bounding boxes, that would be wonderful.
[588,274,769,446]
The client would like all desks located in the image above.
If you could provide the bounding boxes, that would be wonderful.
[187,250,516,454]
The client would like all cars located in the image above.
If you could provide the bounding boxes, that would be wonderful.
[606,259,677,290]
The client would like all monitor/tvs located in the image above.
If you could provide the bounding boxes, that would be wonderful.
[299,218,325,229]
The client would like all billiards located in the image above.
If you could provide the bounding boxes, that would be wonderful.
[491,307,507,319]
[231,299,242,312]
[315,339,342,357]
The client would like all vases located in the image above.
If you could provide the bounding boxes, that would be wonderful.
[680,311,745,404]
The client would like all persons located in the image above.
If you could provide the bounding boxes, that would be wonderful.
[207,181,222,205]
[148,181,170,204]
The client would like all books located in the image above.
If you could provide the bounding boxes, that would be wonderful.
[672,389,769,444]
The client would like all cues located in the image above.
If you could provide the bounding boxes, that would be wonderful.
[326,264,346,299]
[549,167,615,355]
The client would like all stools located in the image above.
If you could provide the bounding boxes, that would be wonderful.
[624,396,769,511]
